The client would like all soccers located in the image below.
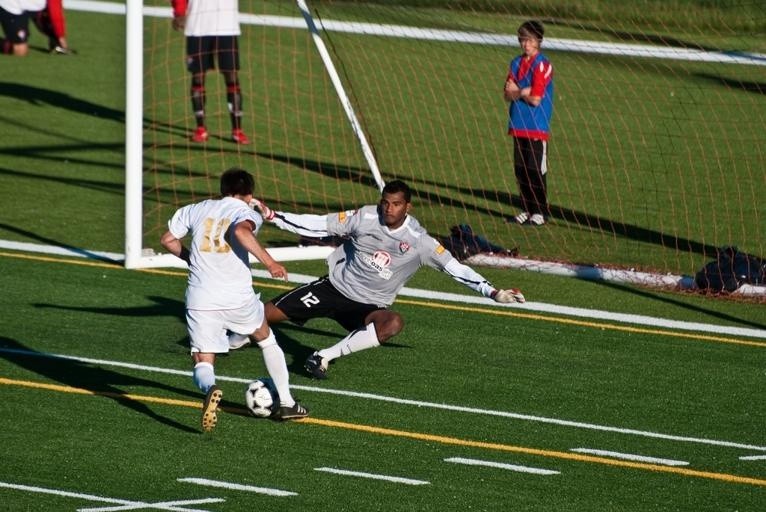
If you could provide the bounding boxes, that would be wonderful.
[244,378,280,418]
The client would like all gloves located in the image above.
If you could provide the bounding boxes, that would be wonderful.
[491,287,525,304]
[247,198,276,222]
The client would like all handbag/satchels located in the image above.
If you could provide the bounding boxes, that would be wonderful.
[696,246,765,296]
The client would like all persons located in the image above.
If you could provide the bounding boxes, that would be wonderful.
[503,21,554,225]
[1,1,68,56]
[224,179,527,377]
[157,166,313,433]
[170,0,250,144]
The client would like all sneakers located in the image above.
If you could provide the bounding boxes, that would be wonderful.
[279,399,310,419]
[192,126,208,143]
[510,212,549,227]
[51,47,76,55]
[200,384,224,432]
[303,350,328,380]
[230,127,250,145]
